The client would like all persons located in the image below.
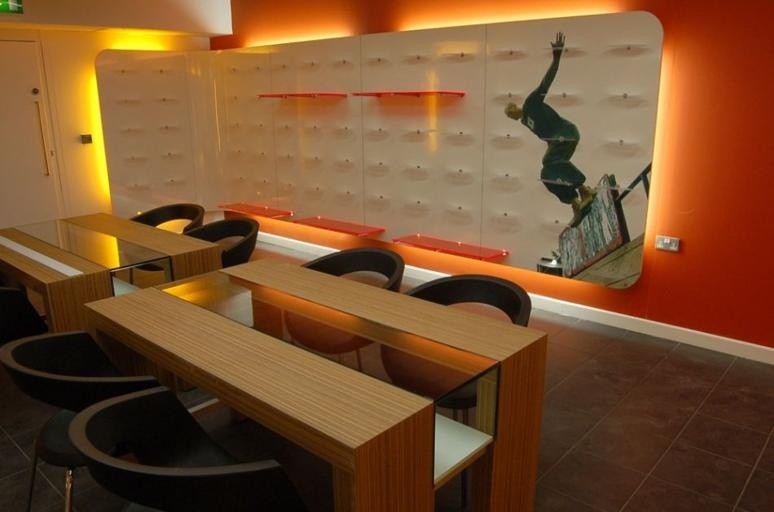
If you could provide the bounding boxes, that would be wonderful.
[506,33,594,227]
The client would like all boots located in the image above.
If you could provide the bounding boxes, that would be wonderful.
[570,183,598,229]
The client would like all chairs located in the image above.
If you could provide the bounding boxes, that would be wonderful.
[0,330,161,512]
[122,202,204,284]
[382,273,531,508]
[0,276,50,346]
[183,217,260,267]
[283,246,405,374]
[68,387,308,512]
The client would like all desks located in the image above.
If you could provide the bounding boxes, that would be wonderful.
[83,257,548,512]
[1,212,221,333]
